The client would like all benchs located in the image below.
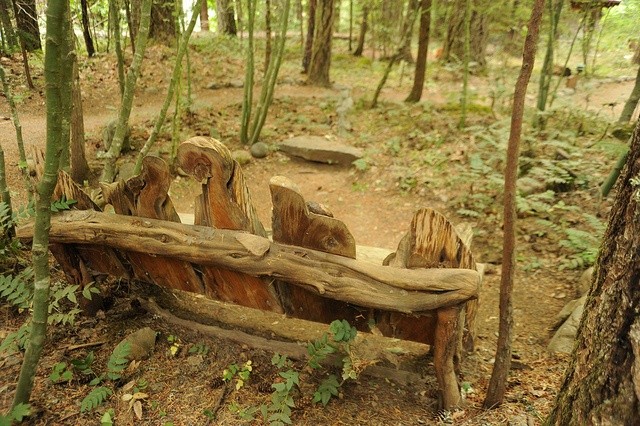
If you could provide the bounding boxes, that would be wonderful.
[13,135,488,418]
